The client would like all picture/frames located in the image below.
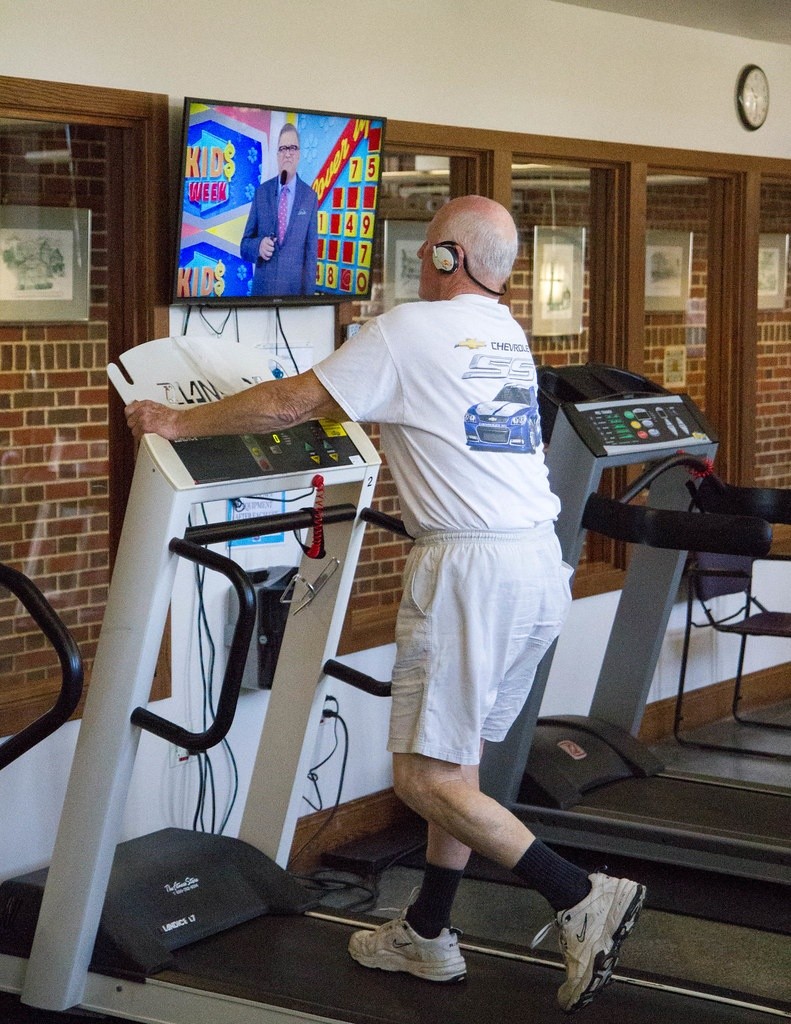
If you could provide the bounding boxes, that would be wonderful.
[644,229,695,313]
[756,232,791,313]
[528,224,587,337]
[381,213,432,314]
[0,202,92,325]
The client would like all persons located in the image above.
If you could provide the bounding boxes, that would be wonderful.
[240,123,318,296]
[124,195,646,1014]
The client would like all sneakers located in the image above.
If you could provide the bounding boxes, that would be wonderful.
[348,887,467,982]
[529,873,650,1012]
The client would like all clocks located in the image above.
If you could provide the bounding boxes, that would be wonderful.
[735,64,769,132]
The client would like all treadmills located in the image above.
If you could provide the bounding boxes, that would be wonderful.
[0,333,791,1024]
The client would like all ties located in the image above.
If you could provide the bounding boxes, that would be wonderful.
[277,186,288,245]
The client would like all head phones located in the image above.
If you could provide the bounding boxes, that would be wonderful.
[432,241,507,296]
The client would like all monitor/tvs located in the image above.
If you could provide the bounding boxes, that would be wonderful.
[169,96,387,308]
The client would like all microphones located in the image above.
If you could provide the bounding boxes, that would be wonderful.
[256,169,287,268]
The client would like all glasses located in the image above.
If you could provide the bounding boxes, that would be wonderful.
[278,145,300,152]
